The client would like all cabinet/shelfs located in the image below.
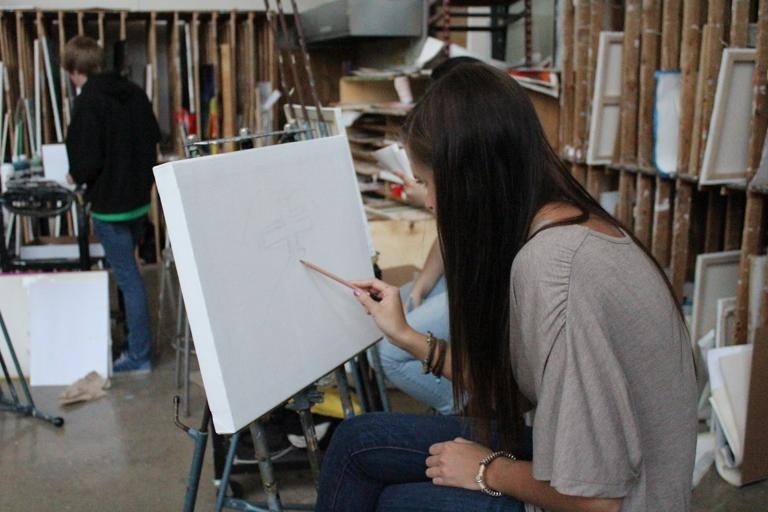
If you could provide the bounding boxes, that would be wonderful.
[333,104,430,214]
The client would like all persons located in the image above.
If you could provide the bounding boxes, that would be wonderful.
[365,55,487,418]
[312,63,699,511]
[61,33,158,375]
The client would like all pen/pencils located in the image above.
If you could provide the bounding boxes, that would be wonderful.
[300,259,371,296]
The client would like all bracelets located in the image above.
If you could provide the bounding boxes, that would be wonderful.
[422,330,447,385]
[475,449,516,497]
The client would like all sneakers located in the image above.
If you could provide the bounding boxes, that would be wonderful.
[113,352,152,375]
